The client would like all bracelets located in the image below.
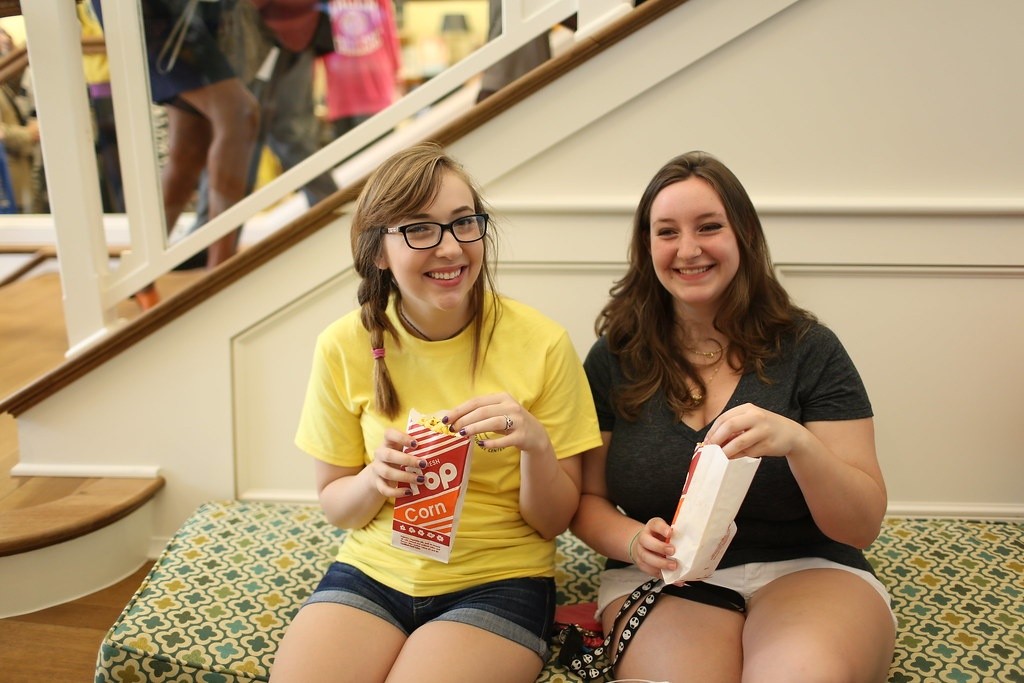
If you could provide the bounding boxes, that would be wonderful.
[628,528,642,566]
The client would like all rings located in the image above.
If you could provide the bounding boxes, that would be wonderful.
[503,415,512,432]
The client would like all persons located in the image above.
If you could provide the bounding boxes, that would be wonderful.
[569,153,900,683]
[0,0,404,269]
[268,140,605,683]
[476,0,576,106]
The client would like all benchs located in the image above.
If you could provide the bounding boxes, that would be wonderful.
[94,501,1024,683]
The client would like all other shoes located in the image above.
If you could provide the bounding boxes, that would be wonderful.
[171,249,207,271]
[134,286,160,312]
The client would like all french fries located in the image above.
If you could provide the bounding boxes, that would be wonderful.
[413,416,459,435]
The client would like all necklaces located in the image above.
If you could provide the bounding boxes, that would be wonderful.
[683,342,729,358]
[688,353,727,401]
[400,301,477,342]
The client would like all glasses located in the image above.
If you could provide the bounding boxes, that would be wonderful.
[380,213,491,251]
